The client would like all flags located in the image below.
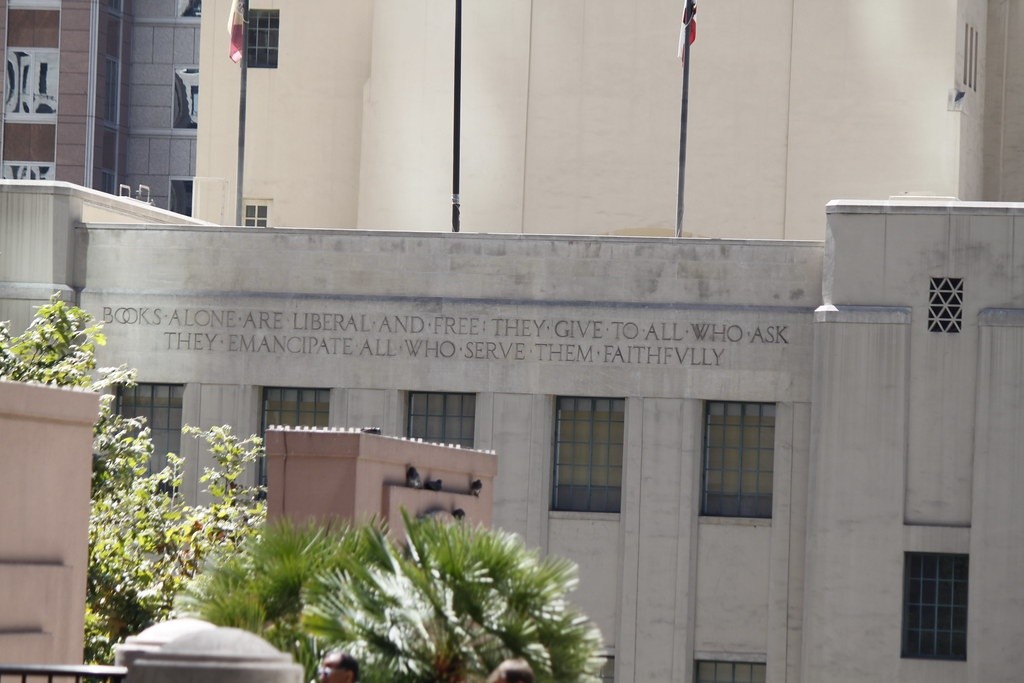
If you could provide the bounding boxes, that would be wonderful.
[226,0,246,64]
[676,0,698,64]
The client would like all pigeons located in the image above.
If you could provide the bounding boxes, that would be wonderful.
[420,479,443,491]
[406,467,423,489]
[452,508,466,521]
[470,477,482,496]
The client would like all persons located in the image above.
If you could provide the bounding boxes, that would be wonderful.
[485,656,535,683]
[316,650,361,683]
